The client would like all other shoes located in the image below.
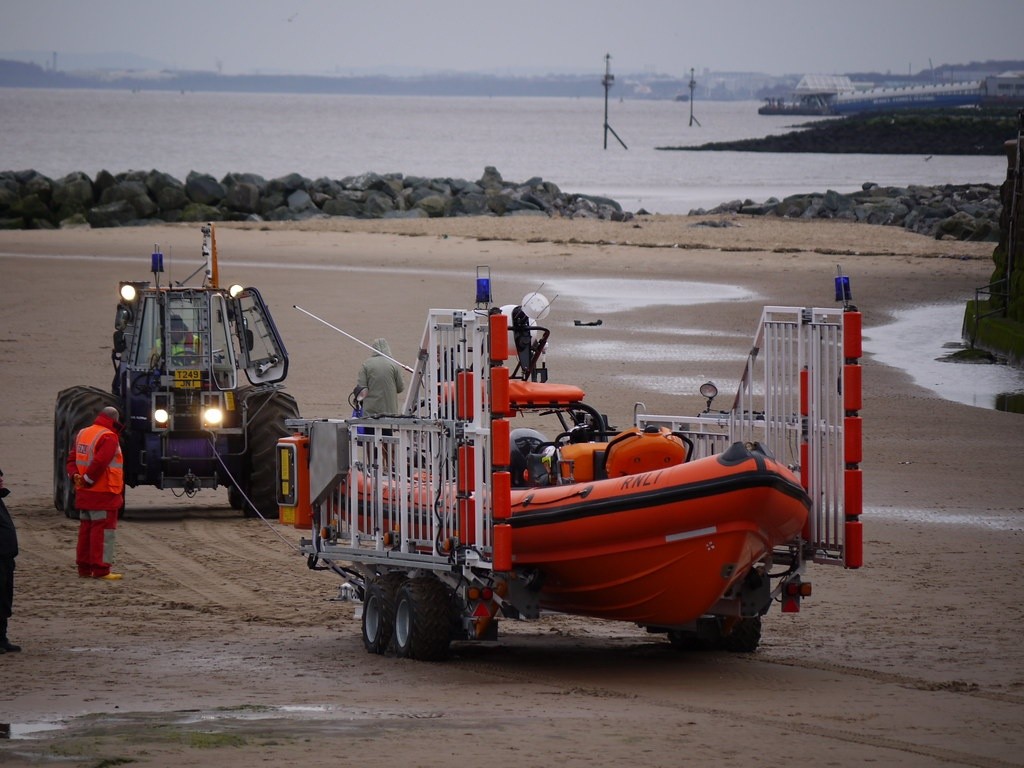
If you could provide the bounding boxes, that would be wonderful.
[0,643,21,654]
[99,572,123,580]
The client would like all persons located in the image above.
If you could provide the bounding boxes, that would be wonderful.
[67,406,124,580]
[146,314,202,368]
[358,338,405,480]
[0,470,21,653]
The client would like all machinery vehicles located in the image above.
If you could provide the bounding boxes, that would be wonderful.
[51,218,307,524]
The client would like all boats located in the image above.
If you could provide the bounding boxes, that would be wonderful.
[319,279,811,628]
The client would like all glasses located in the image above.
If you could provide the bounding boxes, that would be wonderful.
[0,471,3,477]
